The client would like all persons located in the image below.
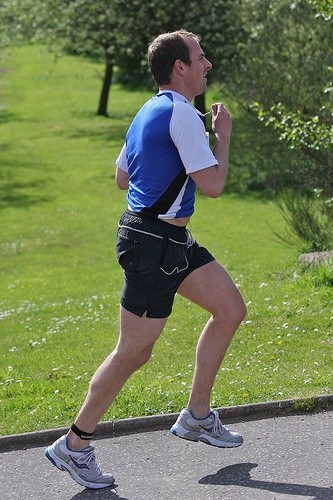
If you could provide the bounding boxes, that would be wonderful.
[44,29,247,490]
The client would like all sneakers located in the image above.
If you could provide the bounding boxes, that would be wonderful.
[169,407,244,449]
[45,434,115,489]
